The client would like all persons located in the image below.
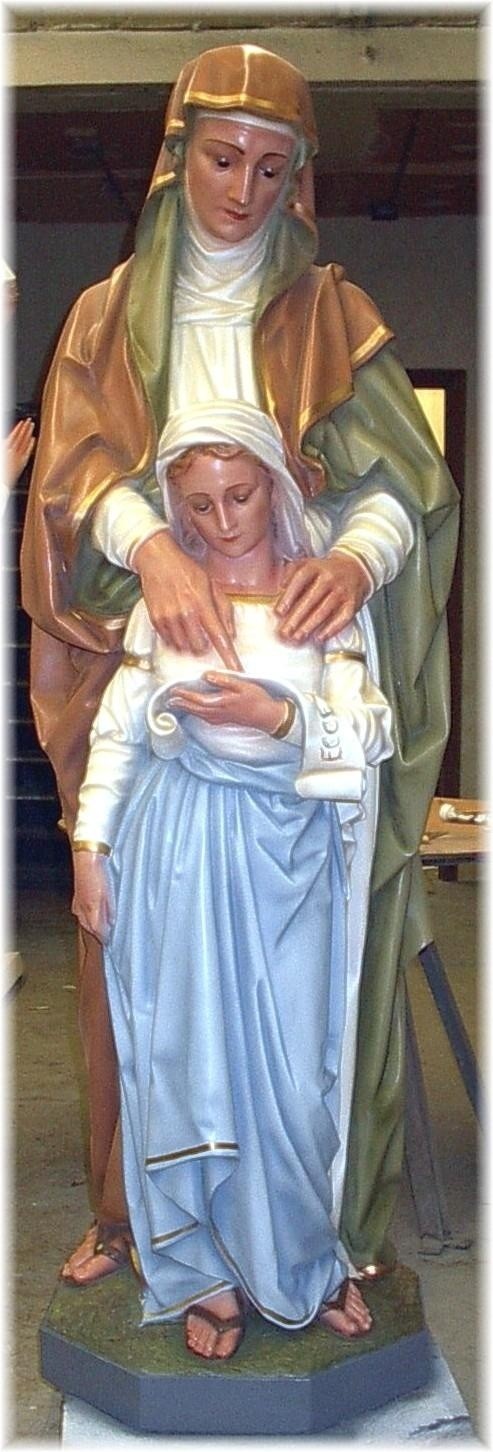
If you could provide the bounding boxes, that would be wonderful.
[66,403,400,1365]
[0,255,38,528]
[16,41,461,1284]
